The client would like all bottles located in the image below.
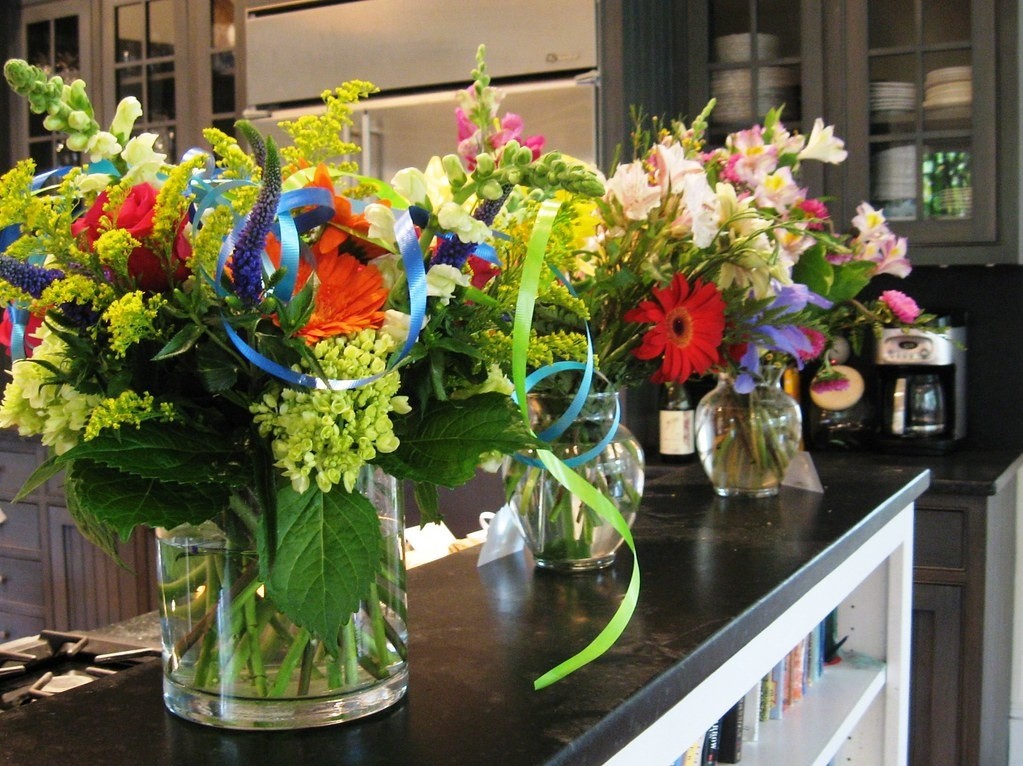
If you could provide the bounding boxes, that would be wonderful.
[656,375,699,464]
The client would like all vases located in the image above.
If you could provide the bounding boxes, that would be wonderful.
[509,393,645,571]
[153,463,413,729]
[691,364,802,497]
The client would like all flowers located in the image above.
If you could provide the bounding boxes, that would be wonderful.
[0,55,965,626]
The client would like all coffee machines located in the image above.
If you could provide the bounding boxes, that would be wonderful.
[866,311,975,459]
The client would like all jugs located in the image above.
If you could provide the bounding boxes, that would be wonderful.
[885,373,945,436]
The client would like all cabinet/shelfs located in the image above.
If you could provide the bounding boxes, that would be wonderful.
[691,1,1022,265]
[644,446,1023,766]
[0,464,933,766]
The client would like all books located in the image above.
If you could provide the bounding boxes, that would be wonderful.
[666,628,823,766]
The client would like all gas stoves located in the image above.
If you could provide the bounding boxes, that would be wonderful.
[0,629,163,717]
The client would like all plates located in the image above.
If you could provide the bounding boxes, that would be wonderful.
[868,81,916,113]
[870,144,932,221]
[922,65,973,107]
[710,31,792,125]
[936,186,974,221]
